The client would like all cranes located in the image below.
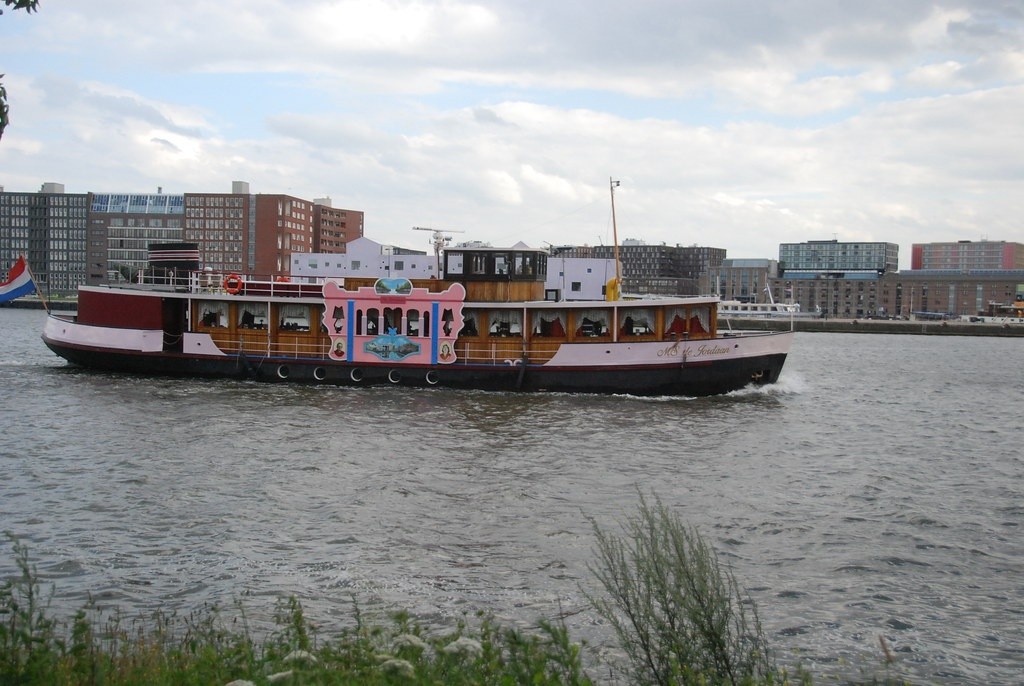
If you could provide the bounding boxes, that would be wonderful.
[411,227,465,255]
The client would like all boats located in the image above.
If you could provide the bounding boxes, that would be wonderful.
[717,299,822,319]
[38,239,793,407]
[956,299,1024,325]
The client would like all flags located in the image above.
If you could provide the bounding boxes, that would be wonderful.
[0,256,36,305]
